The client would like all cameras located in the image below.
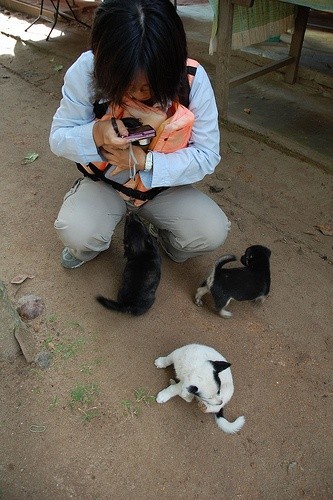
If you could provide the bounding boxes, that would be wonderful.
[121,124,156,146]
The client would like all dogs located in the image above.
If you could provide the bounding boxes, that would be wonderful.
[153,344,246,434]
[95,211,162,316]
[194,244,270,319]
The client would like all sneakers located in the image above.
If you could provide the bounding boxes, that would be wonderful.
[148,223,190,263]
[60,248,86,269]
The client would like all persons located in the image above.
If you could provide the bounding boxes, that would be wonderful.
[49,0,230,270]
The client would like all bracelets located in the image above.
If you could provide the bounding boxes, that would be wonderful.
[144,151,153,172]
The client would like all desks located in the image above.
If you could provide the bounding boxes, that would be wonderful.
[213,0,333,122]
